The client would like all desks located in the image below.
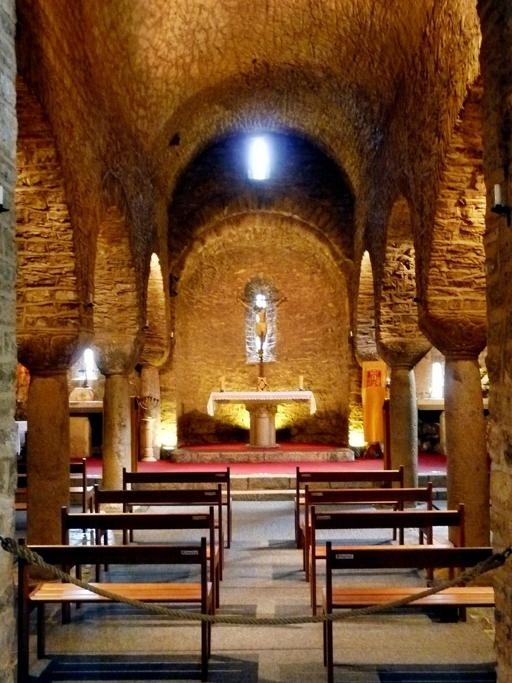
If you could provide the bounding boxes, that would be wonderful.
[208,389,316,449]
[417,399,488,457]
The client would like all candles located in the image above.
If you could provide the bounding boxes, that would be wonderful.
[218,376,224,392]
[298,374,304,390]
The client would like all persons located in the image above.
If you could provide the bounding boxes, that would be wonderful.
[236,293,288,356]
